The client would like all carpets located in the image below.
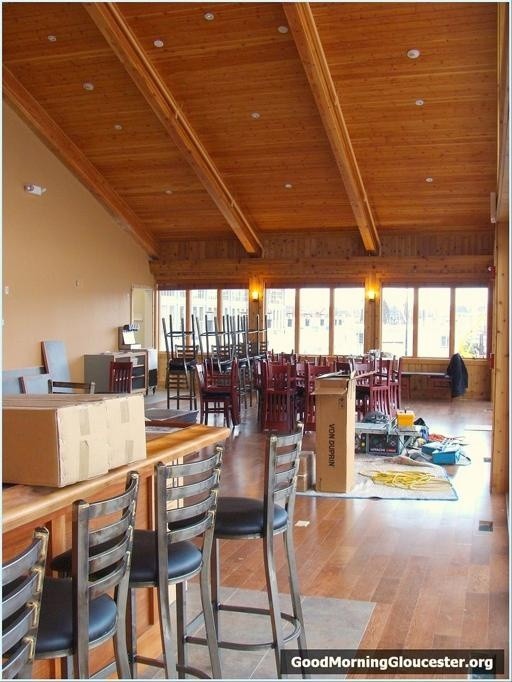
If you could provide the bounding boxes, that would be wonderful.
[294,447,460,503]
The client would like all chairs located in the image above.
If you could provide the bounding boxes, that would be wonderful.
[428,352,466,407]
[2,309,407,681]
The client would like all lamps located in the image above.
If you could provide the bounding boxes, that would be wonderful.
[252,290,259,303]
[368,291,375,302]
